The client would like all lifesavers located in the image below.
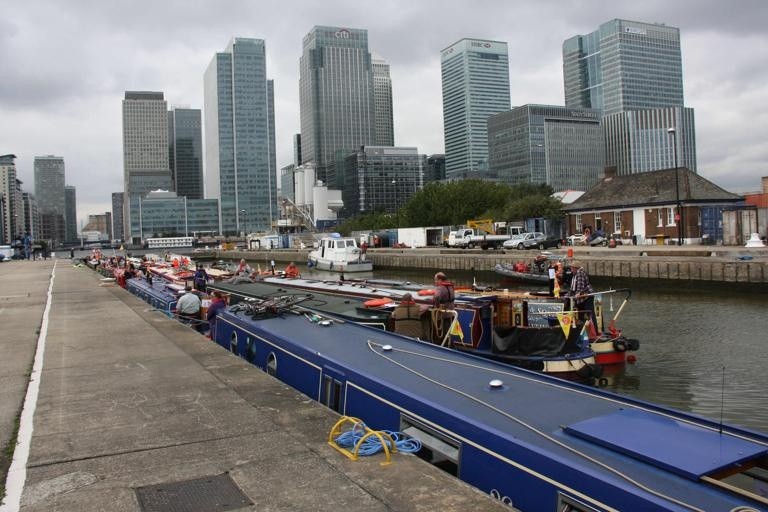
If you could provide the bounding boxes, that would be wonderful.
[551,263,562,277]
[364,298,386,307]
[613,338,629,352]
[418,289,436,297]
[598,378,609,388]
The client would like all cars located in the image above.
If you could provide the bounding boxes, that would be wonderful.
[503,231,585,251]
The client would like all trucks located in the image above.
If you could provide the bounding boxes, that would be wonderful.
[455,229,511,250]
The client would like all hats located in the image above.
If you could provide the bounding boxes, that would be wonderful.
[569,260,583,268]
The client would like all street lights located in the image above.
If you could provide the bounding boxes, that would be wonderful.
[667,127,684,247]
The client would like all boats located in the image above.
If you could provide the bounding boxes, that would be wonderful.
[416,260,639,389]
[307,232,374,273]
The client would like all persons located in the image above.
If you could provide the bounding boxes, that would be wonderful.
[391,293,428,341]
[581,227,592,246]
[571,260,598,335]
[91,249,189,284]
[194,264,210,291]
[284,261,299,278]
[361,241,368,263]
[432,272,455,335]
[176,286,226,341]
[553,260,565,278]
[235,258,251,277]
